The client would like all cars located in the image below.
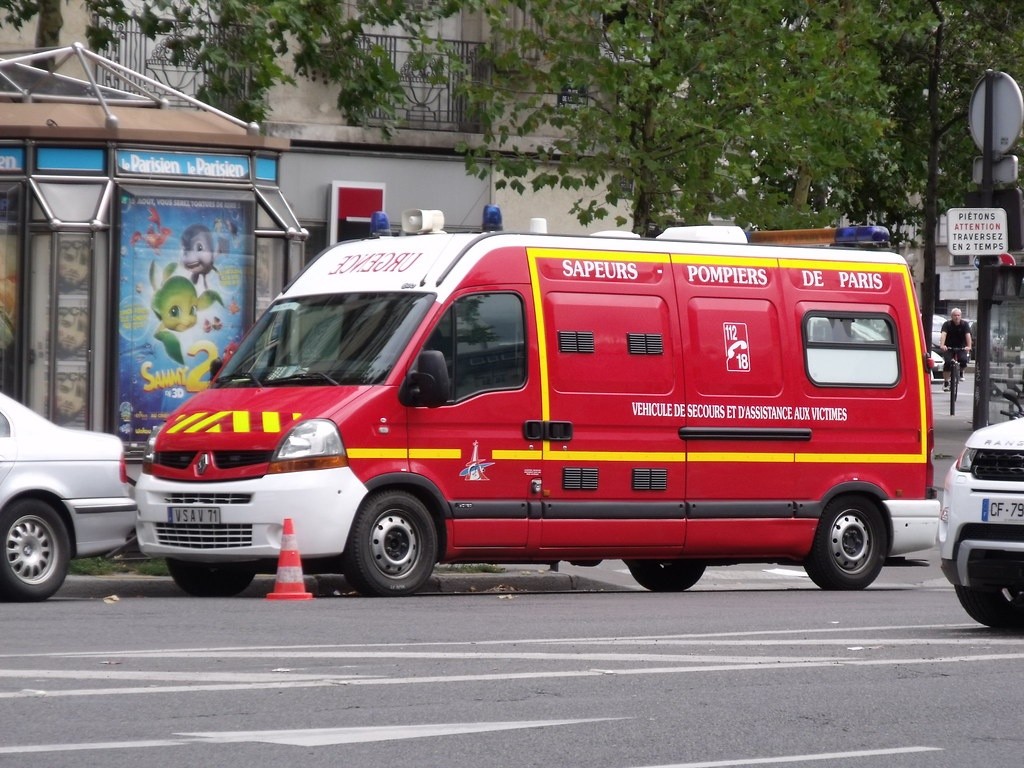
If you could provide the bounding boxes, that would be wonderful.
[935,408,1024,636]
[0,388,132,604]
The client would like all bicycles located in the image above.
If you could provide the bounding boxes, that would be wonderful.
[945,348,975,420]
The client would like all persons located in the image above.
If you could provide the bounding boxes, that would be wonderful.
[940,308,972,392]
[53,372,88,424]
[58,240,91,292]
[57,307,89,360]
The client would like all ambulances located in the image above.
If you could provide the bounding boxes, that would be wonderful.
[133,223,944,595]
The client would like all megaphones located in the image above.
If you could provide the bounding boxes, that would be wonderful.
[402,209,444,234]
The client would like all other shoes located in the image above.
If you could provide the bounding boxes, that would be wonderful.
[943,382,950,391]
[958,370,965,381]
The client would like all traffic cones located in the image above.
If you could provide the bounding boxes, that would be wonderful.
[261,514,311,601]
